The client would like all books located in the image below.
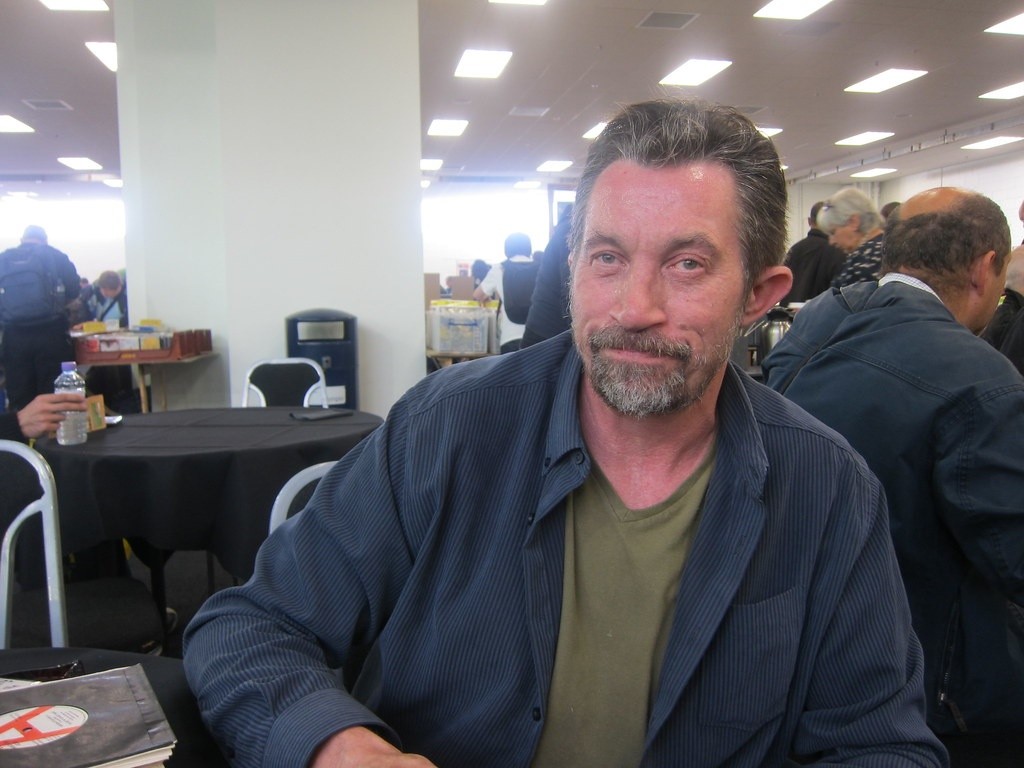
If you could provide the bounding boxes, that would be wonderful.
[0,663,177,768]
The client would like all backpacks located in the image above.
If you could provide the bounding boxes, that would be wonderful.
[502,259,540,324]
[1,244,66,328]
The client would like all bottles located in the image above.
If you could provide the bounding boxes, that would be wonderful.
[762,305,794,355]
[55,361,87,446]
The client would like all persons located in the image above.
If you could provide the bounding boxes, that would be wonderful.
[183,100,951,768]
[765,187,1024,768]
[981,202,1024,374]
[0,227,125,441]
[473,204,574,353]
[779,188,900,316]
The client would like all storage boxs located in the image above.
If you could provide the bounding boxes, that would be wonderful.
[424,297,503,355]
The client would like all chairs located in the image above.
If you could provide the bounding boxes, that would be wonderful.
[0,440,70,651]
[240,357,330,408]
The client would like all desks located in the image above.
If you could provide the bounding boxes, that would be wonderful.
[31,406,382,634]
[0,643,236,768]
[79,351,220,414]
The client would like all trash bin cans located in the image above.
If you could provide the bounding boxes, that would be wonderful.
[286,307,358,410]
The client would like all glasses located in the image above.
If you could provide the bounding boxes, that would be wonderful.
[821,199,836,212]
